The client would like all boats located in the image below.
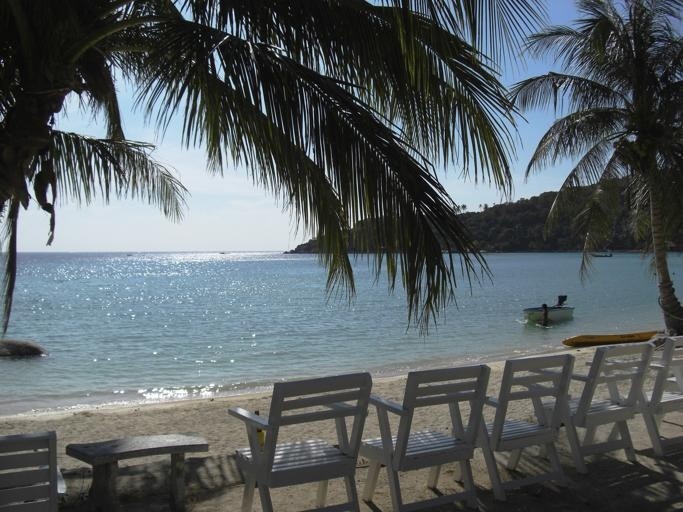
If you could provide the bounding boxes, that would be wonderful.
[561,331,666,347]
[590,253,612,257]
[522,307,575,321]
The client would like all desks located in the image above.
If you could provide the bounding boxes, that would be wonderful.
[66,433,208,512]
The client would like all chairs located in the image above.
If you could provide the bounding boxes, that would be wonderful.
[358,362,493,511]
[448,353,576,501]
[225,371,374,511]
[605,337,682,458]
[0,429,64,512]
[533,342,657,475]
